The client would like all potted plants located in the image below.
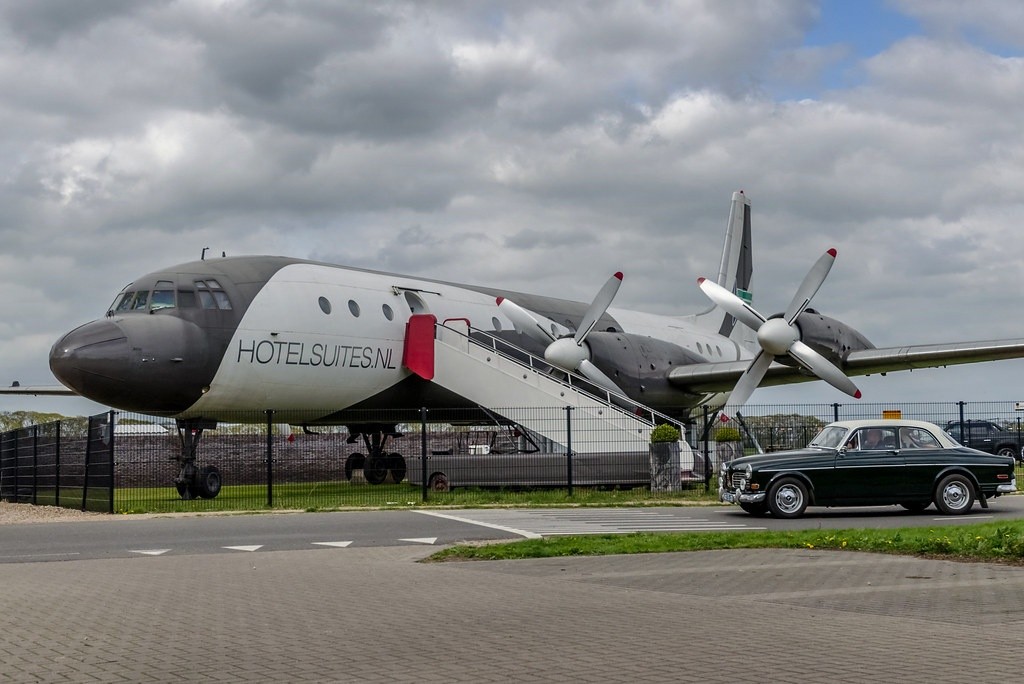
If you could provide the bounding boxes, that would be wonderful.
[715,427,743,477]
[648,423,683,493]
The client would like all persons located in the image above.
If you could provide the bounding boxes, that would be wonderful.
[848,429,886,450]
[902,428,919,448]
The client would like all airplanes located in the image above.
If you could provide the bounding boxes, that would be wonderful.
[47,190,1024,507]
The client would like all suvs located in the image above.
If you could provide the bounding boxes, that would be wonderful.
[942,419,1024,466]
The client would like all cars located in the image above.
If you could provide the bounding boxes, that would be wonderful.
[717,419,1018,519]
